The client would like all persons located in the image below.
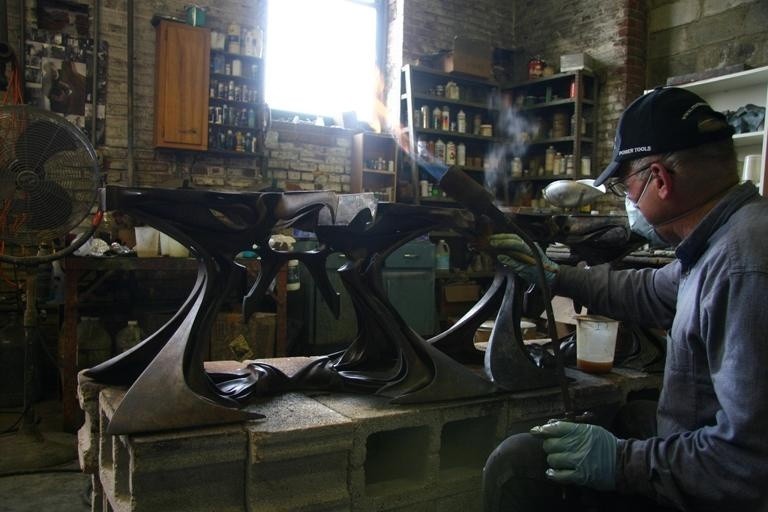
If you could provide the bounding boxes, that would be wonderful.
[479,87,768,511]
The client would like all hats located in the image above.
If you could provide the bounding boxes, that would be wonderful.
[595,85,735,188]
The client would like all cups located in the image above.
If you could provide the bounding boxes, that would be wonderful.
[576,316,619,374]
[160,233,169,256]
[134,225,159,259]
[167,235,189,258]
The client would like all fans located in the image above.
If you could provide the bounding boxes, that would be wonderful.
[1,103,103,474]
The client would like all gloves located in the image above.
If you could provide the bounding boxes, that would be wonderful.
[531,418,619,496]
[493,230,559,286]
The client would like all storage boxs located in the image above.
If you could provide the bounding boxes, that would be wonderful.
[560,53,594,73]
[443,35,493,80]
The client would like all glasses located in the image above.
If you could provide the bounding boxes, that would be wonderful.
[607,161,669,198]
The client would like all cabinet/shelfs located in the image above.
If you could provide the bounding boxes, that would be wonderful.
[400,64,598,208]
[291,240,437,346]
[153,20,266,158]
[643,66,768,197]
[350,132,399,203]
